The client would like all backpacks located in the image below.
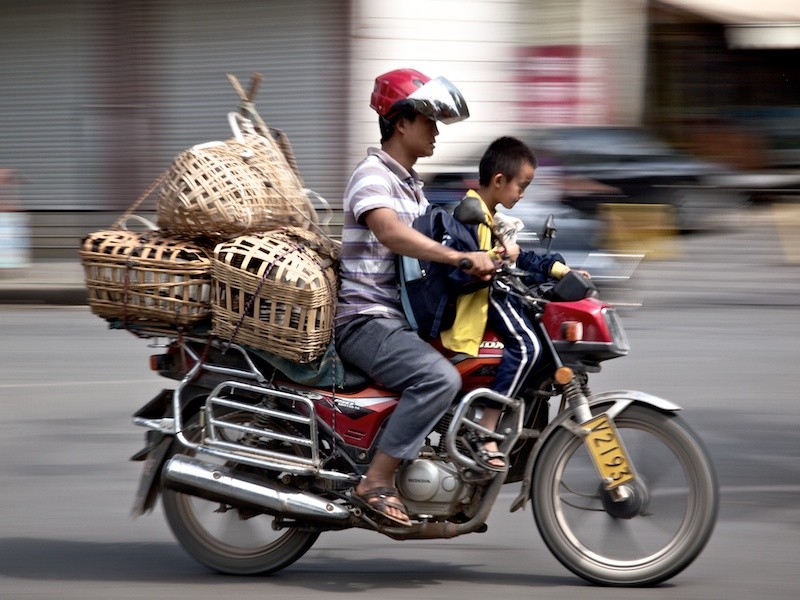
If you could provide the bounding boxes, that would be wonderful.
[397,202,494,338]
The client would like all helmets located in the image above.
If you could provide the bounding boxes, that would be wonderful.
[369,67,470,126]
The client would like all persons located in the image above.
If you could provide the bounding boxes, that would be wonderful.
[331,66,495,526]
[452,136,593,472]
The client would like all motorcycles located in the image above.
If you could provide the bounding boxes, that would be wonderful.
[129,218,719,589]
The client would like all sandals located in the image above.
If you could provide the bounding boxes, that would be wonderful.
[461,427,507,473]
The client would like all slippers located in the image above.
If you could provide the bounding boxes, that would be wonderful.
[350,486,414,527]
